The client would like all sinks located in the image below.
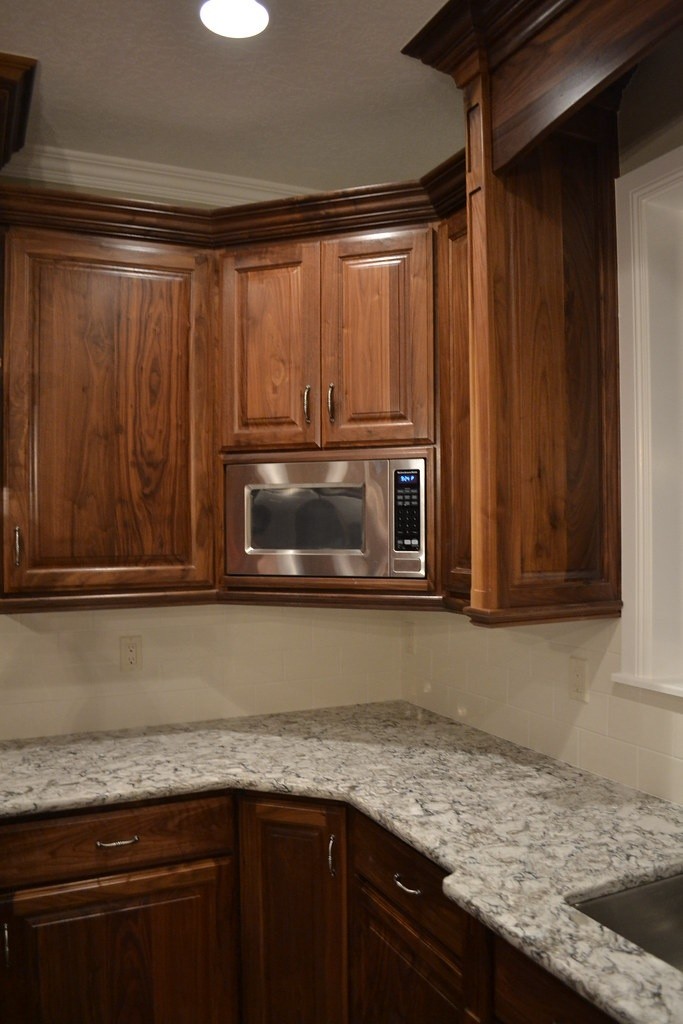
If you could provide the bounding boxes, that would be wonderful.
[563,859,683,973]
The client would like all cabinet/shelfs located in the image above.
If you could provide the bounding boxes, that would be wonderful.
[0,147,214,615]
[351,808,491,1023]
[493,936,620,1023]
[215,177,437,452]
[236,791,349,1024]
[0,794,231,1024]
[435,142,471,614]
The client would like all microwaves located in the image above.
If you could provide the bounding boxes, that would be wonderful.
[225,459,425,577]
